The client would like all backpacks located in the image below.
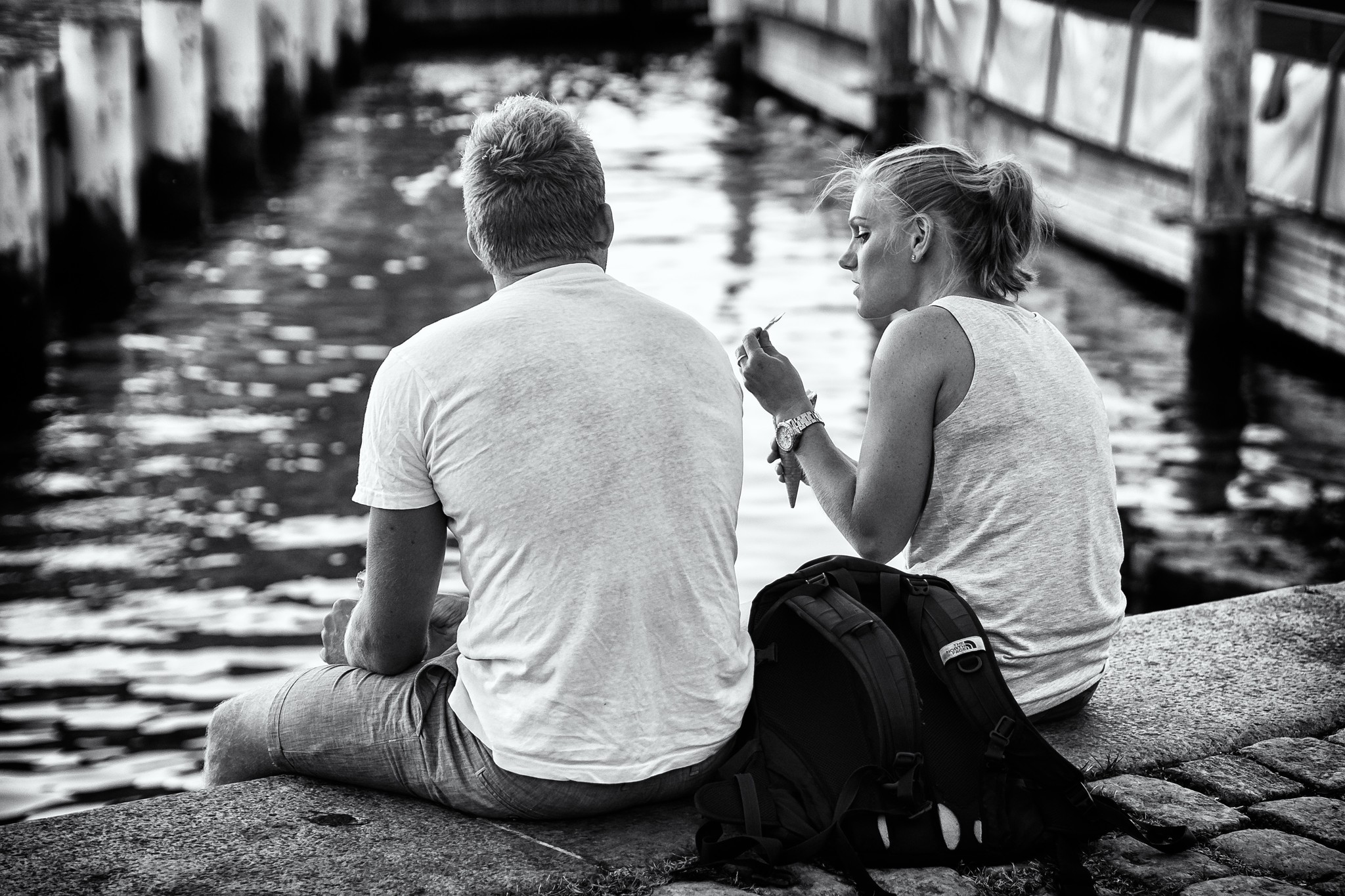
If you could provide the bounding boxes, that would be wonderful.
[749,555,1104,870]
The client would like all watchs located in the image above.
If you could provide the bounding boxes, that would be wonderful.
[775,410,826,453]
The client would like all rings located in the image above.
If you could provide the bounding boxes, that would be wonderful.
[735,353,747,367]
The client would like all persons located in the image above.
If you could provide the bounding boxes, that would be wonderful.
[723,142,1134,733]
[201,89,763,829]
